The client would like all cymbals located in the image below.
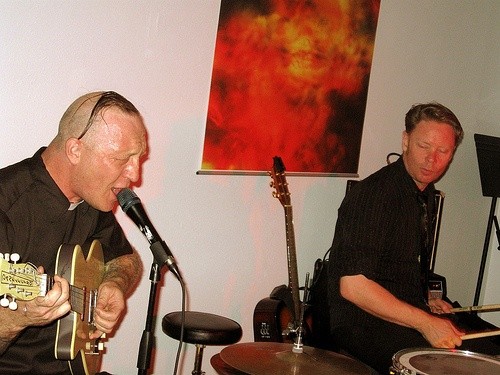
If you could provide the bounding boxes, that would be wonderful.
[210,342,379,375]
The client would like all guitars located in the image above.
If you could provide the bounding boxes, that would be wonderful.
[0,240,106,375]
[252,156,313,343]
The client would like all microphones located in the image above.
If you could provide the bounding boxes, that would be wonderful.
[116,188,181,281]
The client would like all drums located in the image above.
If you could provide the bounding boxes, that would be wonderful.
[390,346,500,375]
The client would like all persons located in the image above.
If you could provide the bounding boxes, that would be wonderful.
[0,91,147,375]
[325,103,466,375]
